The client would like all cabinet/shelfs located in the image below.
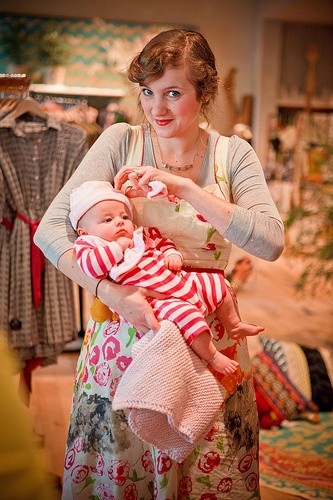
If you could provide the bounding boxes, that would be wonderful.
[279,100,333,184]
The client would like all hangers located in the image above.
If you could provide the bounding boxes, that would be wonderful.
[0,73,49,122]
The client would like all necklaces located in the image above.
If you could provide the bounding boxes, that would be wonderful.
[153,127,202,171]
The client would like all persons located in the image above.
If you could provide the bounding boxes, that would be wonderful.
[33,29,284,500]
[68,179,265,374]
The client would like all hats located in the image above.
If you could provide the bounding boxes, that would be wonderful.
[68,181,133,231]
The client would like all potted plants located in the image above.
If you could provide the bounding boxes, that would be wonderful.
[0,31,31,76]
[41,33,73,85]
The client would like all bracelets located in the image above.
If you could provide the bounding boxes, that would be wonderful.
[96,278,103,298]
